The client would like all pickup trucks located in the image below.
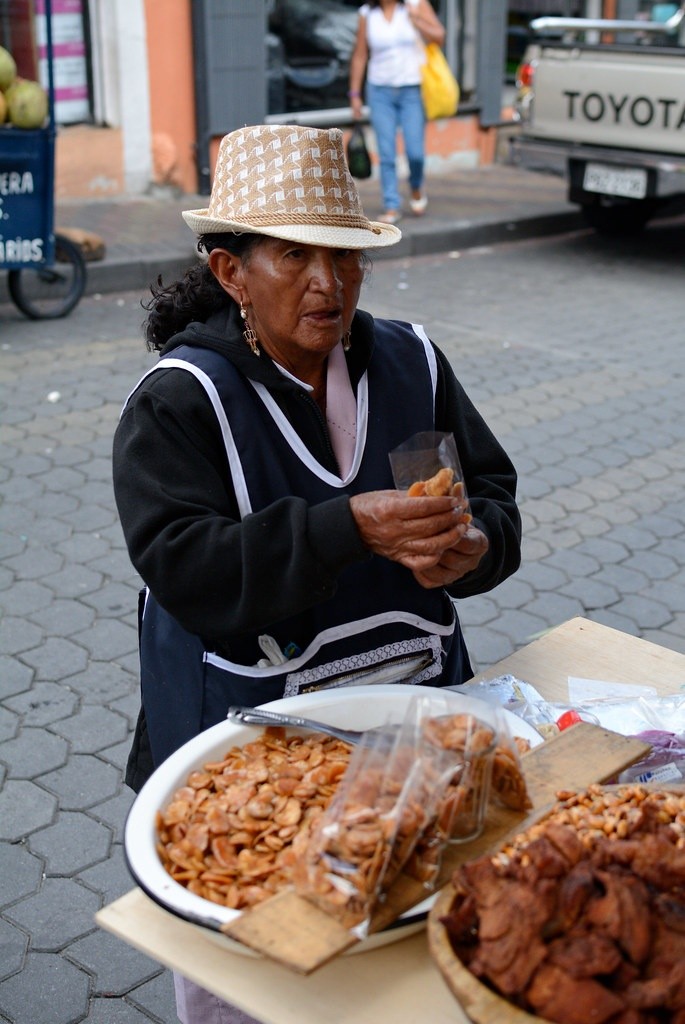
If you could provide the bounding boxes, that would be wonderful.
[496,0,684,239]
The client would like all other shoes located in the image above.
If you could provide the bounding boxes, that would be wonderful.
[377,214,401,224]
[408,189,427,215]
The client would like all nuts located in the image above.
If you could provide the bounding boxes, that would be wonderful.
[407,468,473,528]
[154,713,533,923]
[498,782,685,865]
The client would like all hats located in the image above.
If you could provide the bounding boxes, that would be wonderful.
[182,124,403,249]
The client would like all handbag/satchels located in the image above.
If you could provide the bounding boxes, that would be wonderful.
[419,42,460,120]
[346,120,371,179]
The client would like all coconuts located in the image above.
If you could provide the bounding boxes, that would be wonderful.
[0,46,49,127]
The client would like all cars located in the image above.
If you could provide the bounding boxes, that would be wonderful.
[260,0,379,120]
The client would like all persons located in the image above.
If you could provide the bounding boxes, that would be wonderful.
[112,124,522,795]
[349,0,446,223]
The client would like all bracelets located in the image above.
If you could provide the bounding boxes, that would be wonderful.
[349,90,361,97]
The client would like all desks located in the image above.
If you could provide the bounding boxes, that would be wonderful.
[93,615,685,1024]
